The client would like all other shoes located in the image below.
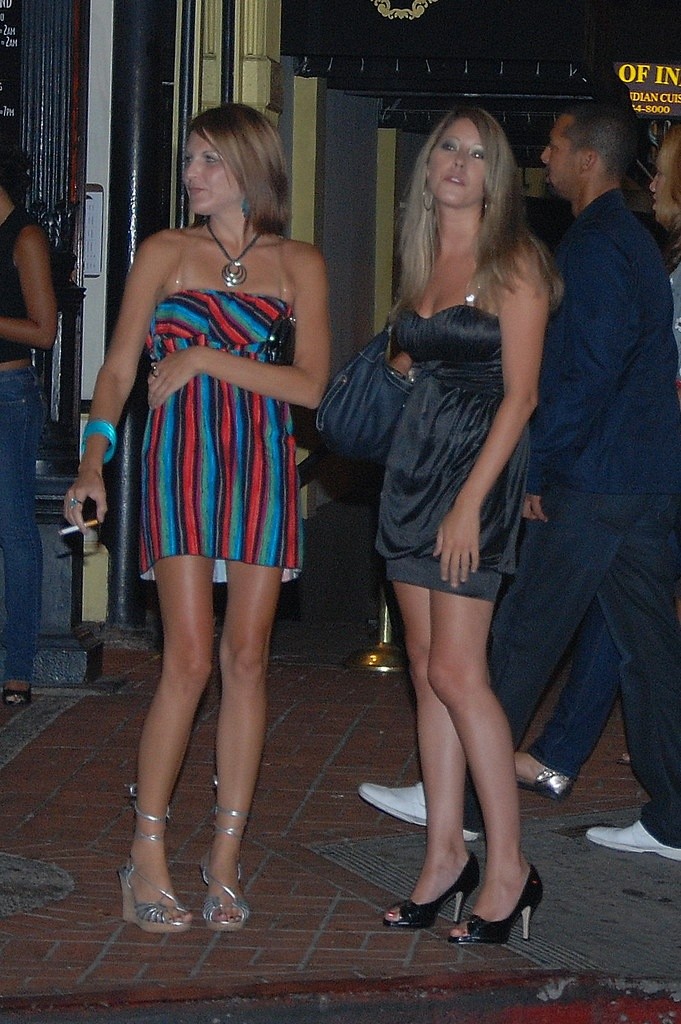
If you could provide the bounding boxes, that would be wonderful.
[516,766,571,800]
[4,684,32,707]
[616,754,633,766]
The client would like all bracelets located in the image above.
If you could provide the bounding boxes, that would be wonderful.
[82,419,117,464]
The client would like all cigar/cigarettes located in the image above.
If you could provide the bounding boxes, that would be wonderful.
[59,519,100,535]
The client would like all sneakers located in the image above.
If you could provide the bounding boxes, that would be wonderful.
[361,782,479,842]
[586,819,681,862]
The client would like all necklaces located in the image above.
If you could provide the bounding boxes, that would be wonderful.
[203,216,261,287]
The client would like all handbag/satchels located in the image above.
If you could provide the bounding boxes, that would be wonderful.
[264,317,298,365]
[315,315,412,465]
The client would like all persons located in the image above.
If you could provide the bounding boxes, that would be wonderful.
[374,100,563,941]
[0,129,57,705]
[356,89,681,859]
[63,103,331,937]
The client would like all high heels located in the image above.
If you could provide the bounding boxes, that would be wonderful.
[117,783,194,934]
[450,866,542,944]
[200,774,249,933]
[383,853,480,928]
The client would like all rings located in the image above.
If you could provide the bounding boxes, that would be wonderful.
[69,498,82,508]
[148,365,158,377]
